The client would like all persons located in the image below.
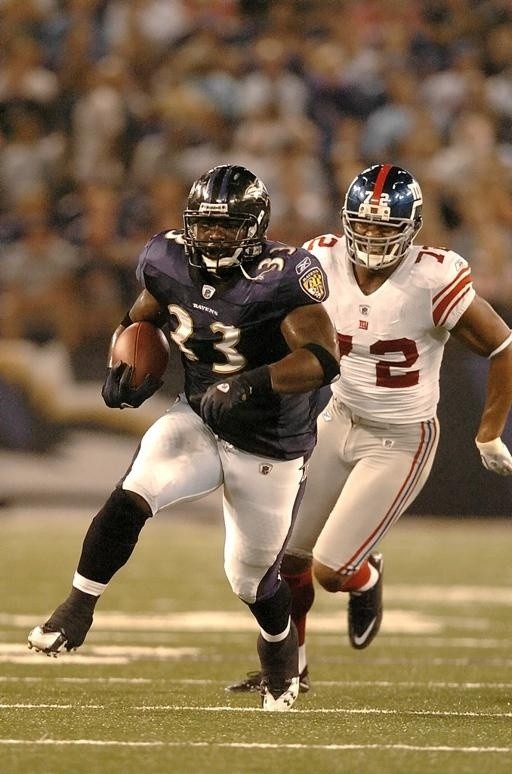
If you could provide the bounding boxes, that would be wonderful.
[26,162,341,712]
[0,0,512,355]
[225,159,511,694]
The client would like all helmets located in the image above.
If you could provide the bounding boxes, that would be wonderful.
[340,164,423,267]
[183,163,268,271]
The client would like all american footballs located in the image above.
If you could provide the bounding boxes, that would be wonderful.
[112,321,171,390]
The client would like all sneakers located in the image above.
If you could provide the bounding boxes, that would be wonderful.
[257,623,299,712]
[223,662,311,696]
[28,603,88,659]
[347,551,383,650]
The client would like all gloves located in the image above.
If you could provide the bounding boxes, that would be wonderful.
[188,364,275,427]
[472,436,512,475]
[101,361,163,410]
